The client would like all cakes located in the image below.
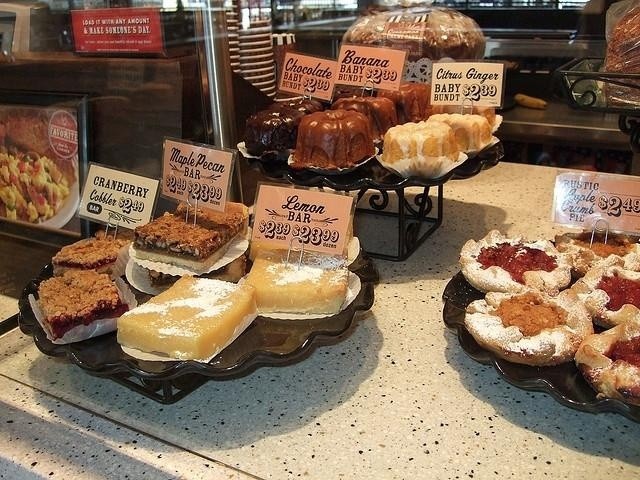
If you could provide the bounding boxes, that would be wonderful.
[239,248,348,315]
[268,95,325,120]
[117,276,258,362]
[377,80,445,121]
[383,120,459,178]
[294,109,375,168]
[52,230,132,272]
[331,96,398,139]
[133,201,248,273]
[250,220,356,265]
[434,101,496,127]
[243,106,301,155]
[37,270,130,337]
[427,113,492,149]
[322,85,371,105]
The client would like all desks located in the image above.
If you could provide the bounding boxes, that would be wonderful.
[469,84,640,171]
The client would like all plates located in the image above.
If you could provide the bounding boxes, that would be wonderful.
[34,153,80,229]
[243,131,507,192]
[16,236,381,388]
[441,240,640,425]
